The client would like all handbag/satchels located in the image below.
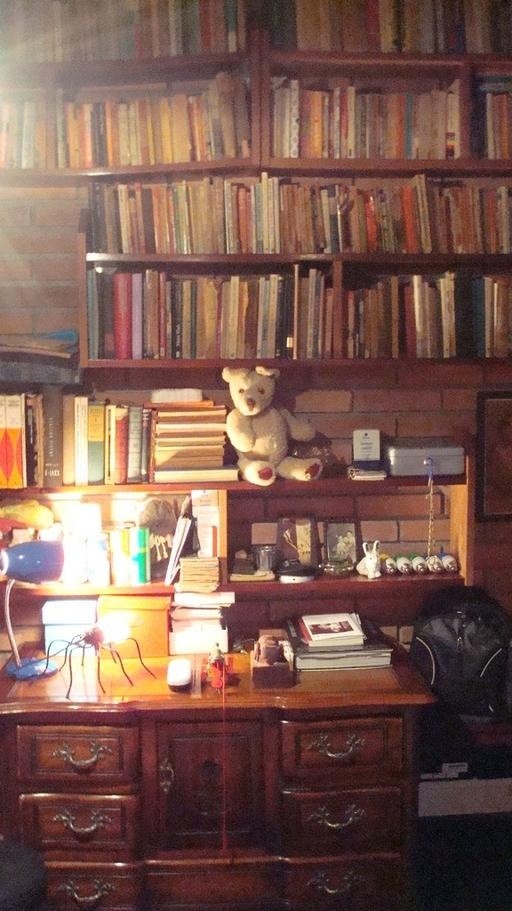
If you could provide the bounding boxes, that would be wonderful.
[411,584,512,717]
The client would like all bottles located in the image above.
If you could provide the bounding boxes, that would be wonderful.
[409,553,427,575]
[442,553,458,572]
[425,554,444,573]
[394,554,412,574]
[381,553,396,574]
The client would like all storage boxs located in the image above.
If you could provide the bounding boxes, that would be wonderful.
[42,599,97,655]
[97,595,171,658]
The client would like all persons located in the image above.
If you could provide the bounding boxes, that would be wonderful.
[335,531,356,560]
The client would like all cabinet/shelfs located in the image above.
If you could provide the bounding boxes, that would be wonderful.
[0,24,512,631]
[0,634,440,911]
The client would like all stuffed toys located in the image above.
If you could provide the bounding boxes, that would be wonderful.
[221,365,324,490]
[356,540,384,580]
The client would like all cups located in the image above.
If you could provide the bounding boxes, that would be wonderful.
[252,545,280,572]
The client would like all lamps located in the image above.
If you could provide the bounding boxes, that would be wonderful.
[0,540,65,678]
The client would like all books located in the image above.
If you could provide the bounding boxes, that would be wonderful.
[0,389,238,489]
[0,0,238,62]
[88,265,512,360]
[1,72,251,170]
[271,77,512,158]
[89,171,512,254]
[170,489,235,653]
[278,611,395,672]
[109,527,148,584]
[275,0,512,56]
[1,332,76,359]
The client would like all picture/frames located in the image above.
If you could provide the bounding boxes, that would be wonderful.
[476,390,512,523]
[323,519,362,564]
